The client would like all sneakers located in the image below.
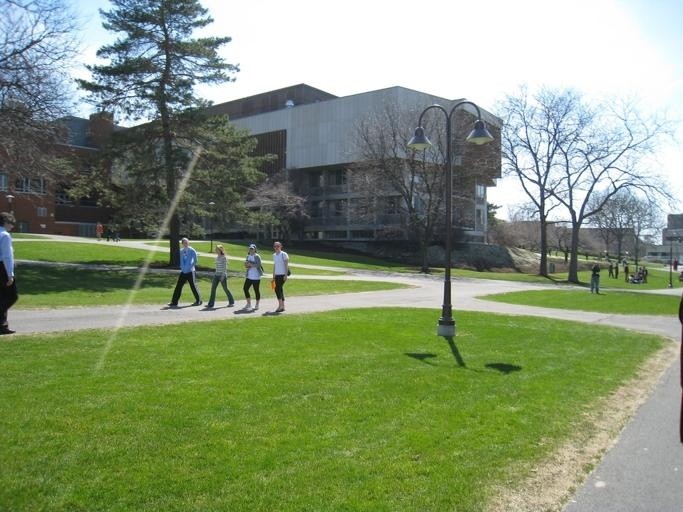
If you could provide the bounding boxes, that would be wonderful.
[168,300,235,307]
[276,306,285,312]
[1,329,15,334]
[243,303,259,310]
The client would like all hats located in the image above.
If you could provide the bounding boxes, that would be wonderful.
[217,245,224,252]
[249,244,256,249]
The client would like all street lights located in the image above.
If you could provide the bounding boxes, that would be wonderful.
[406,100,496,337]
[663,231,683,289]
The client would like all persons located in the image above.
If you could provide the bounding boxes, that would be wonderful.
[203,244,234,307]
[96,222,121,243]
[0,211,17,336]
[166,237,201,306]
[242,244,263,310]
[271,241,289,312]
[590,263,600,295]
[598,249,648,285]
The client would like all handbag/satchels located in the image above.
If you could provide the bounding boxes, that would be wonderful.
[287,268,291,277]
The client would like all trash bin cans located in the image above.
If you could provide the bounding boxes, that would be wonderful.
[549,262,555,273]
[18,220,28,233]
